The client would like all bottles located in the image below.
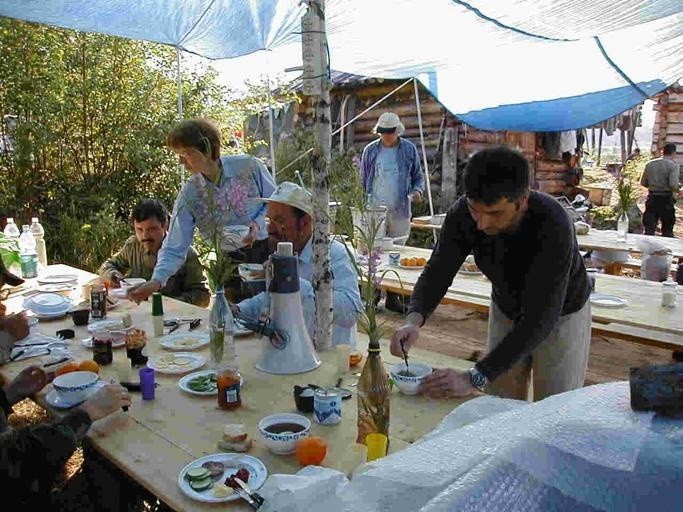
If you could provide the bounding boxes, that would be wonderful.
[4,217,48,290]
[660,274,679,308]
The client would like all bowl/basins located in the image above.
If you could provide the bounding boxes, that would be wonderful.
[389,361,434,395]
[257,413,313,457]
[224,225,250,242]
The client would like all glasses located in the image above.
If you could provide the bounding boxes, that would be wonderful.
[263,214,298,227]
[173,150,192,160]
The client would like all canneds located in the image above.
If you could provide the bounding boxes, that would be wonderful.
[314,388,342,425]
[91,286,107,319]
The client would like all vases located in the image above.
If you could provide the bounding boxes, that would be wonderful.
[615,209,629,244]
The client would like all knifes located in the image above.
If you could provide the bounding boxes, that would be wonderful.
[233,475,264,506]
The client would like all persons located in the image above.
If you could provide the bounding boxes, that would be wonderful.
[624,147,646,166]
[359,112,426,313]
[641,143,683,237]
[97,199,210,309]
[0,252,132,512]
[220,180,363,354]
[576,132,584,157]
[126,119,279,316]
[390,147,592,403]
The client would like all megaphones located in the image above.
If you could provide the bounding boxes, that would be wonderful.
[254,242,322,374]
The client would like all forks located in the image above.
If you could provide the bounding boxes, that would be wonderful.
[233,488,260,511]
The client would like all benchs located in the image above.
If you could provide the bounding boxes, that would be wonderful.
[574,250,678,279]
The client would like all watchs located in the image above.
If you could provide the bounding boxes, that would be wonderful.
[469,367,491,389]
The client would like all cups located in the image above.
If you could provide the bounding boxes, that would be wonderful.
[586,268,597,290]
[342,441,368,481]
[366,433,388,462]
[336,344,350,376]
[407,194,416,202]
[293,385,326,413]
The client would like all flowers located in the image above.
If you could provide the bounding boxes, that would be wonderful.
[187,159,255,361]
[310,149,409,443]
[610,161,637,222]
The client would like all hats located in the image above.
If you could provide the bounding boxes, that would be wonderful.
[372,111,406,138]
[254,180,314,219]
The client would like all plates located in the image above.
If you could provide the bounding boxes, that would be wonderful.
[589,293,627,307]
[12,262,262,410]
[335,387,352,401]
[329,202,484,276]
[177,452,268,503]
